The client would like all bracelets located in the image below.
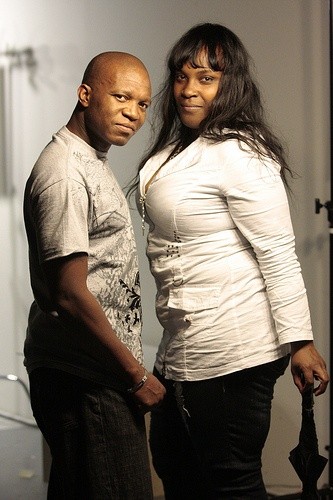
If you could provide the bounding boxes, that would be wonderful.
[127,368,148,392]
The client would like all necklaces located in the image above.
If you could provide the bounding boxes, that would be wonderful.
[137,139,183,237]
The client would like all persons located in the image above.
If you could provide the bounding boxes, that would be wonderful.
[23,51,168,500]
[123,23,331,500]
